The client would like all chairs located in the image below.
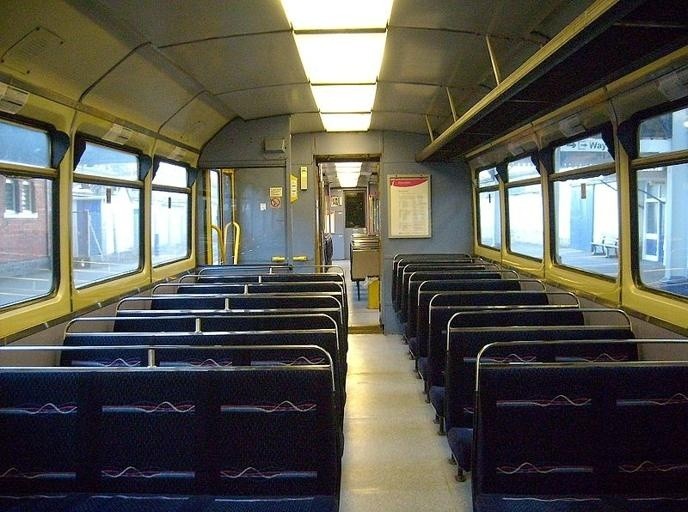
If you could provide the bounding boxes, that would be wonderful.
[589,235,619,257]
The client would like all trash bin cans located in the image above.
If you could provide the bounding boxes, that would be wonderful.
[367,276,380,308]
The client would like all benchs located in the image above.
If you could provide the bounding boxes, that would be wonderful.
[1,264,349,512]
[392,251,687,510]
[349,232,381,301]
